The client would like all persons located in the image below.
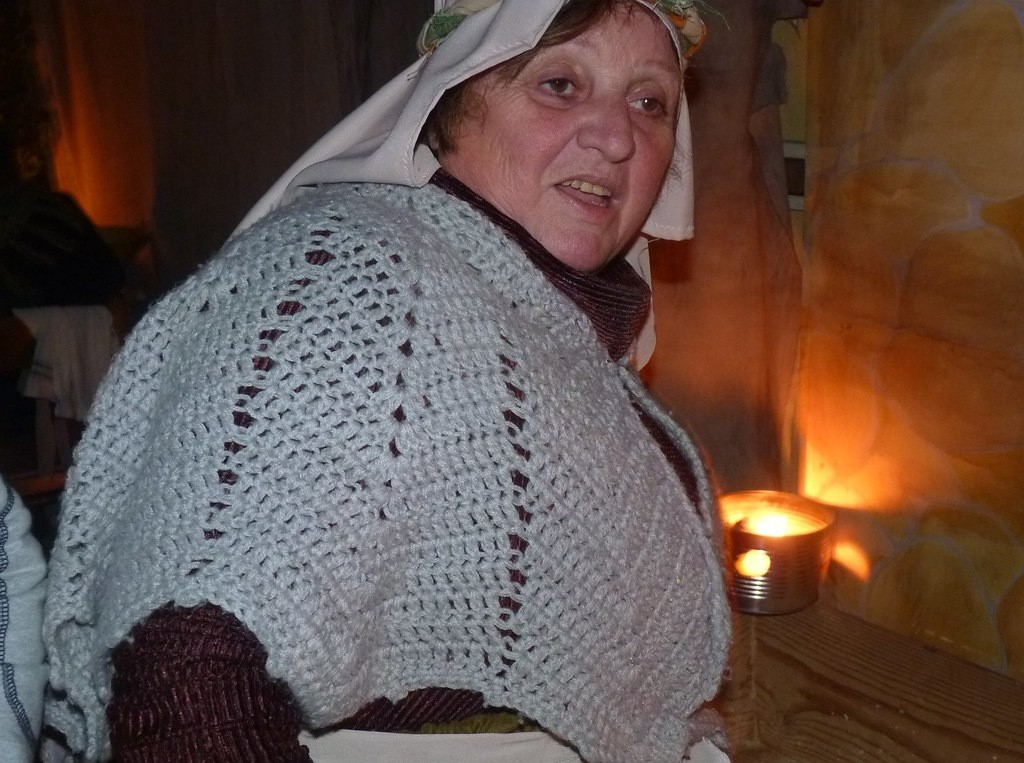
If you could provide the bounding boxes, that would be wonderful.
[46,0,734,763]
[0,0,116,311]
